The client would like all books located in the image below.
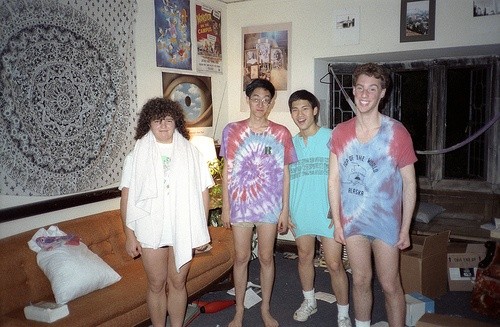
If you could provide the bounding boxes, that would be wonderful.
[24,300,70,323]
[149,303,200,327]
[480,223,495,231]
[227,280,262,309]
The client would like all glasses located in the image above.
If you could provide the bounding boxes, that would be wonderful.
[249,96,271,104]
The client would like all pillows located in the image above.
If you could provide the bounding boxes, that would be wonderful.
[409,189,500,244]
[28,226,121,305]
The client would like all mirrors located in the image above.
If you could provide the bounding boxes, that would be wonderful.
[412,200,446,223]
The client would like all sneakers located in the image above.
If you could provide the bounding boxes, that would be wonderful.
[292,294,318,322]
[337,313,352,327]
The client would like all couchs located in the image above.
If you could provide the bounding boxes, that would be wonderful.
[0,208,235,327]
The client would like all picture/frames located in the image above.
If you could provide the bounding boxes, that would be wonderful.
[400,0,435,42]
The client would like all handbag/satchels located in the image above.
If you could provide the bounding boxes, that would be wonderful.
[468,240,499,317]
[195,289,236,313]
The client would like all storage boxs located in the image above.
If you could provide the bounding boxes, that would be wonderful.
[448,244,489,291]
[403,293,435,327]
[397,232,447,301]
[414,313,493,327]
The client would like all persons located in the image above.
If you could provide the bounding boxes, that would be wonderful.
[328,62,417,327]
[221,79,290,327]
[121,97,210,327]
[283,90,352,327]
